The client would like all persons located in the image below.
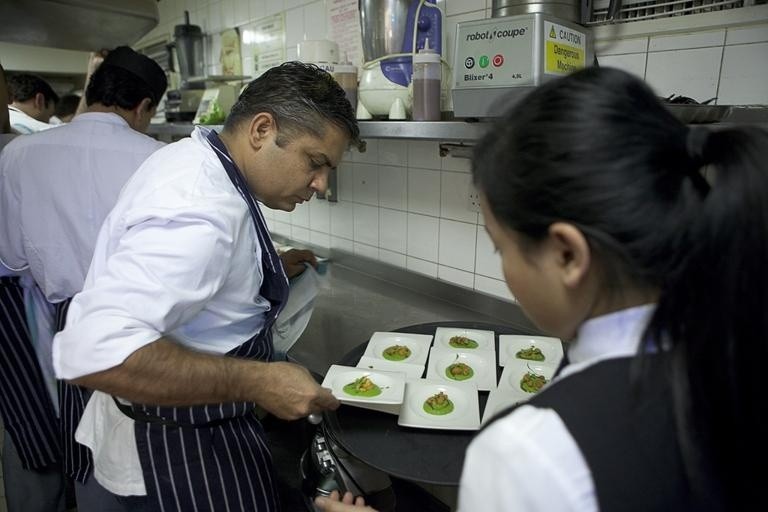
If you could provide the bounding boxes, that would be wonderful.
[312,64,768,512]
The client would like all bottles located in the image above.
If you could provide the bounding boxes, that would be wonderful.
[408,35,446,121]
[332,49,357,114]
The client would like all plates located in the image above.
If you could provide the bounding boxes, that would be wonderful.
[317,322,572,438]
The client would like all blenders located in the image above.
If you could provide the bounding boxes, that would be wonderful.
[162,7,209,123]
[354,0,453,121]
